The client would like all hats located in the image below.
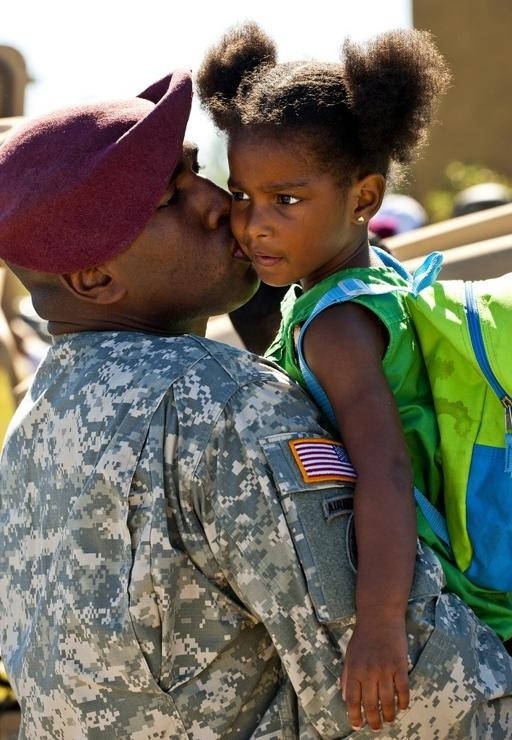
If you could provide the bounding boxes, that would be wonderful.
[1,69,192,275]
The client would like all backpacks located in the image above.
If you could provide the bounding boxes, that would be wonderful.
[297,245,512,643]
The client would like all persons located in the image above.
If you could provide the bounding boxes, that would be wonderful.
[0,70,512,740]
[196,23,512,733]
